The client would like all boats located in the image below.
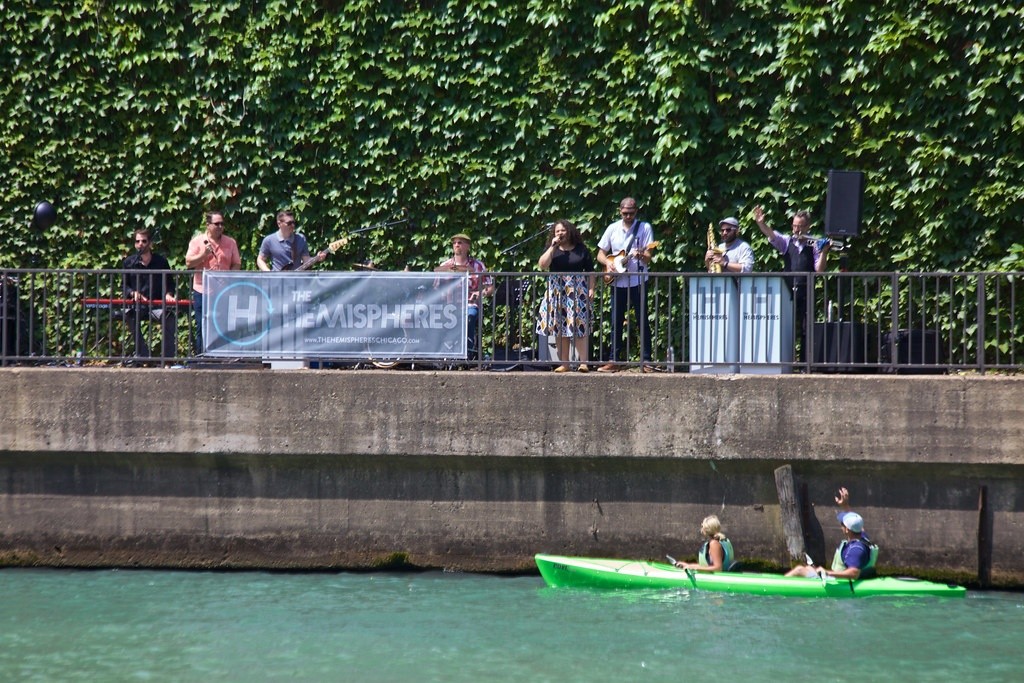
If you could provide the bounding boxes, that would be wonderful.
[534,553,967,599]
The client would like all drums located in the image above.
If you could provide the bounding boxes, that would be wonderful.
[366,318,411,369]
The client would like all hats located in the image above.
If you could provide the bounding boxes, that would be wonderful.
[450,234,471,242]
[837,511,864,533]
[719,217,739,227]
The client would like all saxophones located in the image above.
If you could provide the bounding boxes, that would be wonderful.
[707,222,723,273]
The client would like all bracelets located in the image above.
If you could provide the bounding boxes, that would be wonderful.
[723,261,728,269]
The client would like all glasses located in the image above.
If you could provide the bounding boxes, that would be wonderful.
[282,221,295,226]
[720,228,737,232]
[211,221,224,226]
[452,241,462,245]
[135,239,147,243]
[622,211,635,216]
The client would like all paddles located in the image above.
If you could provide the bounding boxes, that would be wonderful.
[804,553,827,587]
[665,554,695,580]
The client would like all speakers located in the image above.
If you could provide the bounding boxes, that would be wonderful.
[799,321,880,374]
[824,170,865,238]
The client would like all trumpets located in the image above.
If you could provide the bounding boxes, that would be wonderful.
[793,230,852,252]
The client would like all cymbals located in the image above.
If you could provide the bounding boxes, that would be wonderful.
[352,263,379,271]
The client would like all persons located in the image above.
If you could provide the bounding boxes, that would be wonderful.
[752,204,834,374]
[674,515,734,573]
[704,217,754,273]
[597,197,654,373]
[256,211,326,272]
[536,219,596,372]
[186,212,241,358]
[785,487,874,582]
[121,227,177,368]
[432,234,494,370]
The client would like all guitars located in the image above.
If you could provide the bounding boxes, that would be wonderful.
[602,240,661,284]
[295,238,349,272]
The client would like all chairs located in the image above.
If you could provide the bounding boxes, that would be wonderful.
[729,561,744,572]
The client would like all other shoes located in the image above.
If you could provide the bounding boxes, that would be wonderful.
[165,363,186,369]
[643,361,655,373]
[555,364,570,372]
[578,363,589,373]
[597,360,620,372]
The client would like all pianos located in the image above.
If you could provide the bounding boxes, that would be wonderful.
[79,297,194,368]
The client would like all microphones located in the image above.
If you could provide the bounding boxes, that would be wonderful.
[204,240,216,258]
[552,236,562,247]
